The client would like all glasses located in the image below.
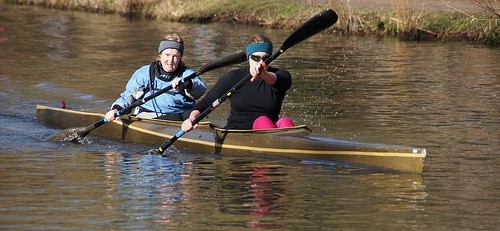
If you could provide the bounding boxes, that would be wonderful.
[250,54,270,62]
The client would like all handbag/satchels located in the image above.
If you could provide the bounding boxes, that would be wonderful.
[153,113,185,121]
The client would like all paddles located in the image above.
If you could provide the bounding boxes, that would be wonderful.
[42,47,249,143]
[134,7,339,156]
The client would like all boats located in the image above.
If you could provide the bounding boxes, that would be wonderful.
[37,104,426,174]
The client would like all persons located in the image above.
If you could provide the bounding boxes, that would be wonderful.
[181,34,294,133]
[104,33,207,120]
